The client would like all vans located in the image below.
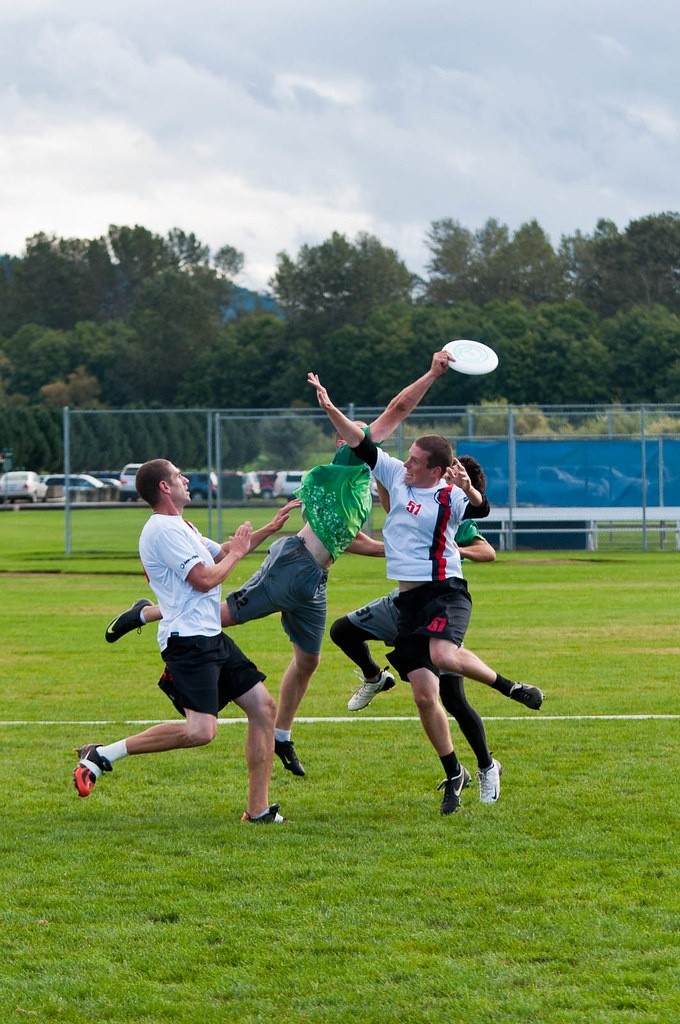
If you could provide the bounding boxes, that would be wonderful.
[121,463,145,501]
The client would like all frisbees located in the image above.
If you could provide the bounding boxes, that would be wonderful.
[442,338,499,376]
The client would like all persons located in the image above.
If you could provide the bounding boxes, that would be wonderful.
[72,350,544,824]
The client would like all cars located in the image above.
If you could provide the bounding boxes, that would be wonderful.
[1,470,48,504]
[482,462,650,503]
[97,477,120,489]
[182,470,311,508]
[45,475,108,498]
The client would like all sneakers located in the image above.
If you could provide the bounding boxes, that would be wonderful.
[437,763,472,816]
[72,744,113,797]
[347,666,396,711]
[241,803,297,825]
[105,599,154,643]
[475,751,502,807]
[509,682,546,710]
[275,738,305,776]
